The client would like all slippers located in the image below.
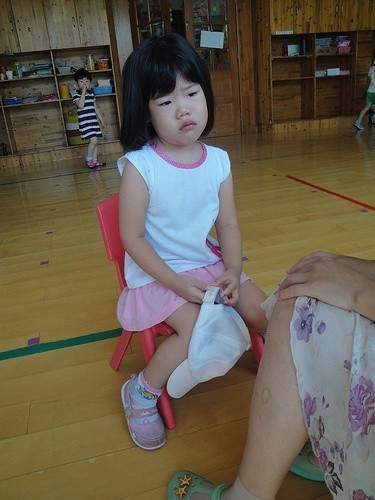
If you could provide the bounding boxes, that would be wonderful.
[287,439,326,481]
[167,470,234,500]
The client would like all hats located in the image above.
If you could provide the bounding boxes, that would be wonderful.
[166,285,251,400]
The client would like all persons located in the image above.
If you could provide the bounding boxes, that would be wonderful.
[72,68,107,169]
[116,37,268,449]
[354,51,375,130]
[166,250,375,500]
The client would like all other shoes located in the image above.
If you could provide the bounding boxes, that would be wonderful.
[354,120,365,130]
[93,159,106,167]
[86,159,97,169]
[371,115,375,124]
[121,373,168,449]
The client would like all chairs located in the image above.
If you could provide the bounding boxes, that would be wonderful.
[95,190,263,429]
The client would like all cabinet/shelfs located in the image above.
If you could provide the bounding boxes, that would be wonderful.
[251,0,375,136]
[0,0,129,163]
